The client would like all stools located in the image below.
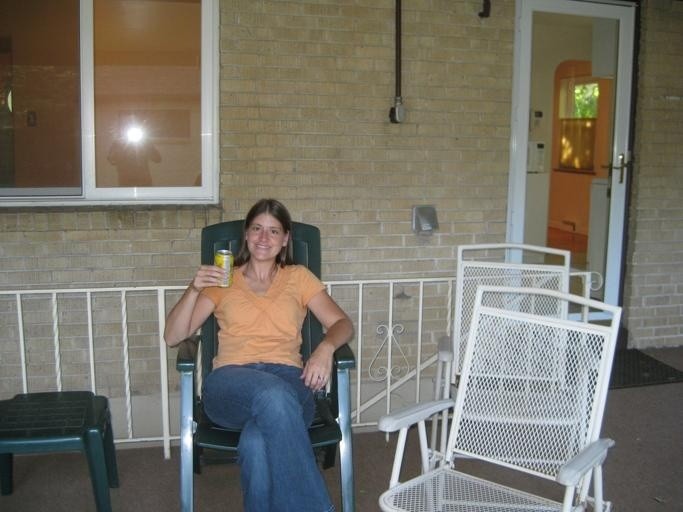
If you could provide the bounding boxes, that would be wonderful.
[0,391,120,512]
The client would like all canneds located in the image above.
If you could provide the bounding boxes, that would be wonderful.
[213,249,234,289]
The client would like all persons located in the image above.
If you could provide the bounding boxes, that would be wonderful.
[108,111,164,188]
[163,196,356,512]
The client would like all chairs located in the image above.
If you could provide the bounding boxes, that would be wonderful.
[175,218,357,511]
[379,285,625,510]
[430,244,571,469]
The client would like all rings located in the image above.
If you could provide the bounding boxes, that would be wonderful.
[317,376,323,381]
